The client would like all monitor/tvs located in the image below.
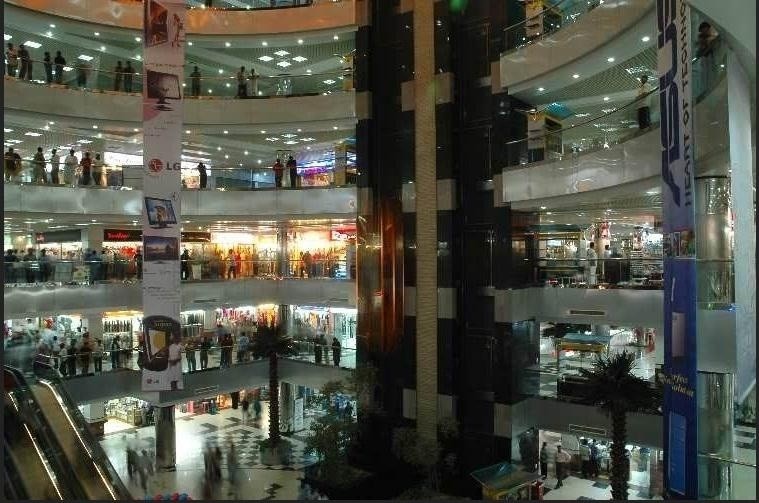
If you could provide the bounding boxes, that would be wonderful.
[143,197,178,230]
[141,236,180,264]
[144,323,168,362]
[146,0,171,48]
[145,70,182,104]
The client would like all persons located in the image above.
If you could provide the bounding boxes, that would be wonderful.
[540,442,547,479]
[199,336,211,369]
[32,147,103,185]
[580,439,599,479]
[4,147,20,183]
[190,66,201,96]
[168,334,183,390]
[184,340,196,372]
[111,335,131,368]
[637,74,651,128]
[332,337,340,366]
[553,445,571,489]
[181,249,191,279]
[236,332,249,362]
[5,248,142,282]
[314,335,321,364]
[587,242,597,285]
[198,162,208,189]
[697,22,719,49]
[226,249,237,279]
[252,249,259,275]
[273,159,285,187]
[33,327,104,376]
[300,251,312,278]
[286,156,297,188]
[114,60,136,91]
[6,43,33,79]
[220,332,234,367]
[44,51,66,83]
[237,66,259,96]
